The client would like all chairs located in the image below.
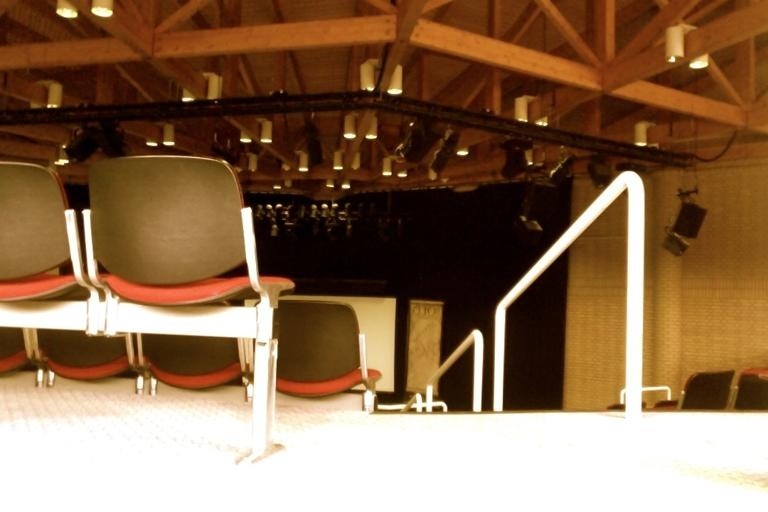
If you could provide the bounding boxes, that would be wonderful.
[249,293,386,414]
[82,144,311,406]
[2,150,108,398]
[19,272,149,403]
[132,281,259,410]
[1,324,41,394]
[653,366,739,411]
[730,362,767,412]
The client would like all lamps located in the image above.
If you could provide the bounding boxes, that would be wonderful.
[515,91,577,190]
[322,53,471,191]
[145,72,309,195]
[648,13,725,82]
[51,1,129,33]
[252,198,306,235]
[312,202,353,233]
[590,122,648,183]
[22,74,80,117]
[658,167,709,257]
